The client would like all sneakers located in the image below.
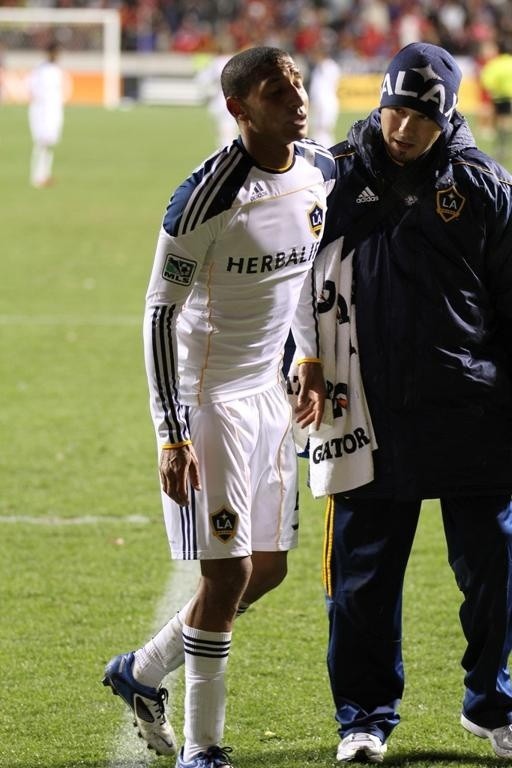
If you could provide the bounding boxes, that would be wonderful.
[335,732,387,763]
[460,711,512,761]
[99,650,179,757]
[175,745,240,768]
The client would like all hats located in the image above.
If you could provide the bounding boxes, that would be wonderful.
[376,39,463,134]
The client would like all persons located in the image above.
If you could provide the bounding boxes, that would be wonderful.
[278,37,512,765]
[104,46,340,767]
